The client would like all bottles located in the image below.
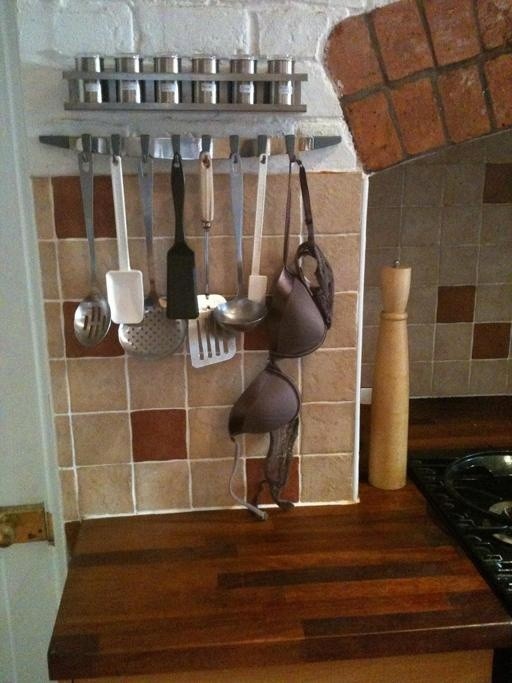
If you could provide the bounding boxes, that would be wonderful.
[229,59,258,104]
[154,56,182,103]
[267,59,296,105]
[75,56,104,104]
[114,56,144,103]
[192,56,219,104]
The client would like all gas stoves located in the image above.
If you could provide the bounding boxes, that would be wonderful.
[406,444,512,619]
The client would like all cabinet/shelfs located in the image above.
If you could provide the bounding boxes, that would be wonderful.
[47,478,512,683]
